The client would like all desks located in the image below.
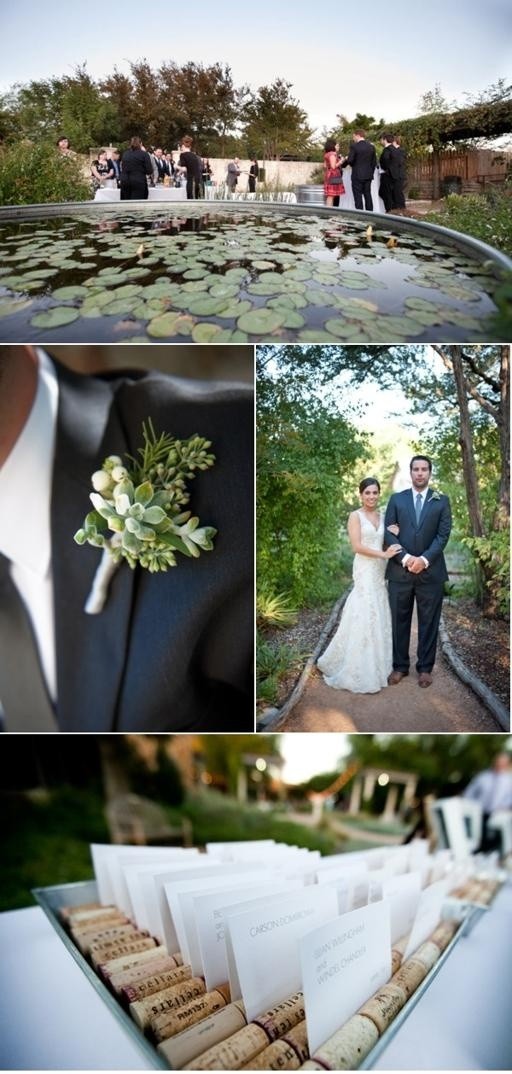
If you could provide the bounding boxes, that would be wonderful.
[0,865,511,1071]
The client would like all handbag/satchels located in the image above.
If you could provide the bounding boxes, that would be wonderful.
[331,176,342,185]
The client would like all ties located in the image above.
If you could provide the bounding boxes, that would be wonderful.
[415,494,422,527]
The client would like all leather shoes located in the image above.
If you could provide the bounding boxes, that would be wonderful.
[388,671,409,685]
[419,672,432,688]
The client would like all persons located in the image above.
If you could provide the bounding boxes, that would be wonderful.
[385,456,451,690]
[322,140,346,206]
[463,750,512,854]
[377,133,400,212]
[0,346,252,734]
[345,129,376,211]
[58,136,214,201]
[226,156,242,198]
[316,478,401,695]
[244,158,258,192]
[393,138,407,208]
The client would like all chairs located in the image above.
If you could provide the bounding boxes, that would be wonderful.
[104,792,193,847]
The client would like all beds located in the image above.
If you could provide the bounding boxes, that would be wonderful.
[93,185,216,201]
